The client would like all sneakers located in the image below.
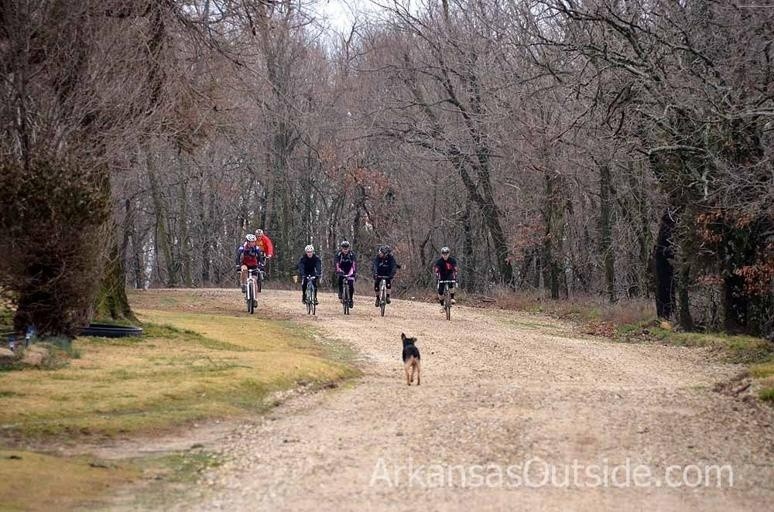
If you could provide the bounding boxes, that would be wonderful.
[338,292,341,299]
[315,298,318,303]
[241,284,246,293]
[302,296,305,303]
[350,301,352,308]
[451,299,455,304]
[375,298,380,306]
[440,306,444,312]
[252,300,257,307]
[386,297,390,303]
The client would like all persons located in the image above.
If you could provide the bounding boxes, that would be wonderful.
[234,233,264,308]
[254,228,274,281]
[298,244,322,305]
[371,245,397,308]
[433,247,458,313]
[333,240,358,309]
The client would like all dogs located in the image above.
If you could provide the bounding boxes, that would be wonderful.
[400,333,422,386]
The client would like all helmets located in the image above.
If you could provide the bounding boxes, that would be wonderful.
[381,246,391,253]
[341,241,349,246]
[442,247,450,253]
[305,245,314,251]
[247,234,256,241]
[255,229,263,237]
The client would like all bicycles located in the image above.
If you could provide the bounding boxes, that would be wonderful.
[336,273,356,314]
[244,255,268,314]
[376,274,390,316]
[302,275,320,315]
[440,281,454,321]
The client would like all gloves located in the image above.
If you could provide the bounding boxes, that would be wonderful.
[236,265,241,271]
[260,266,264,271]
